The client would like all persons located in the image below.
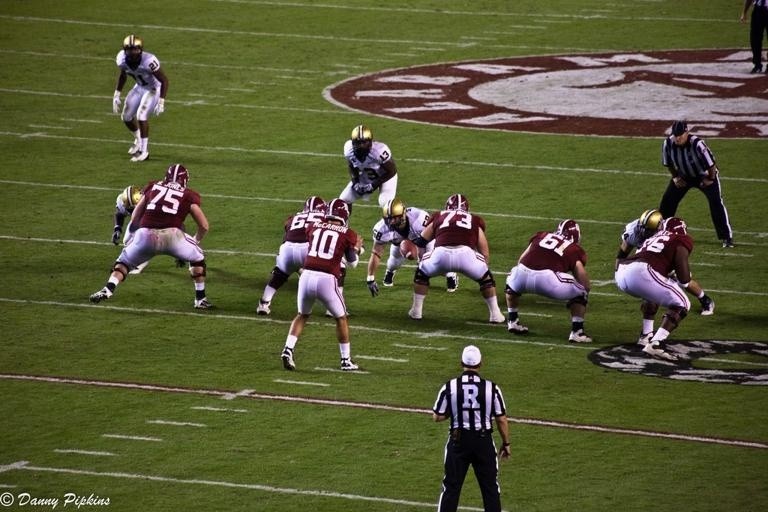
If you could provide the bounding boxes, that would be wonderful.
[87,164,217,310]
[657,119,734,249]
[111,185,187,275]
[503,219,593,343]
[111,34,168,163]
[281,198,359,371]
[613,209,679,283]
[408,193,505,324]
[338,124,398,211]
[742,1,768,74]
[256,196,350,318]
[432,345,511,512]
[367,198,459,298]
[614,209,715,363]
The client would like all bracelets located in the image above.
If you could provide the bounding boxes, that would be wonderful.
[708,176,715,181]
[502,442,511,446]
[367,274,375,282]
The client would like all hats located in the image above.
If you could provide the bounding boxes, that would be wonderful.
[672,120,687,137]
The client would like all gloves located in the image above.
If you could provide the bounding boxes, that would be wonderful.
[154,103,164,117]
[499,443,511,462]
[112,231,122,245]
[353,183,372,195]
[113,96,122,113]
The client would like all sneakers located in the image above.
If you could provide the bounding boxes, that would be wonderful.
[89,286,114,304]
[699,291,715,316]
[194,297,212,309]
[130,152,149,162]
[723,239,734,248]
[128,143,141,154]
[129,261,149,274]
[751,67,761,73]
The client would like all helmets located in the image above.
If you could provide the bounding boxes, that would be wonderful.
[165,164,188,187]
[122,186,144,213]
[123,35,143,59]
[352,125,373,155]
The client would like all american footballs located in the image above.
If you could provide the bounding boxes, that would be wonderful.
[400,240,417,259]
